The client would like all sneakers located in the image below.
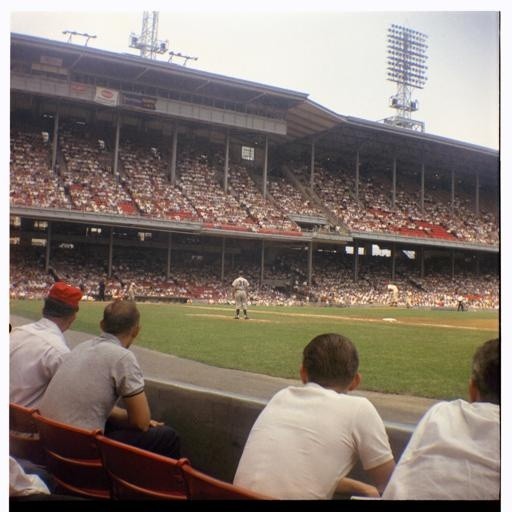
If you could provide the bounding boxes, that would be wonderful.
[234,315,249,319]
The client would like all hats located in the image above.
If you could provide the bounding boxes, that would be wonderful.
[239,270,245,276]
[47,282,83,309]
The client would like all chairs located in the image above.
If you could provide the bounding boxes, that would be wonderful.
[90,428,188,498]
[29,410,113,511]
[177,454,274,500]
[9,402,42,468]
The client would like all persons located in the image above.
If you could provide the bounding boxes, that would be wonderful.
[36,299,181,481]
[9,243,499,308]
[456,295,464,312]
[231,332,396,501]
[10,282,86,413]
[376,337,501,501]
[290,152,498,245]
[230,270,250,319]
[10,124,322,231]
